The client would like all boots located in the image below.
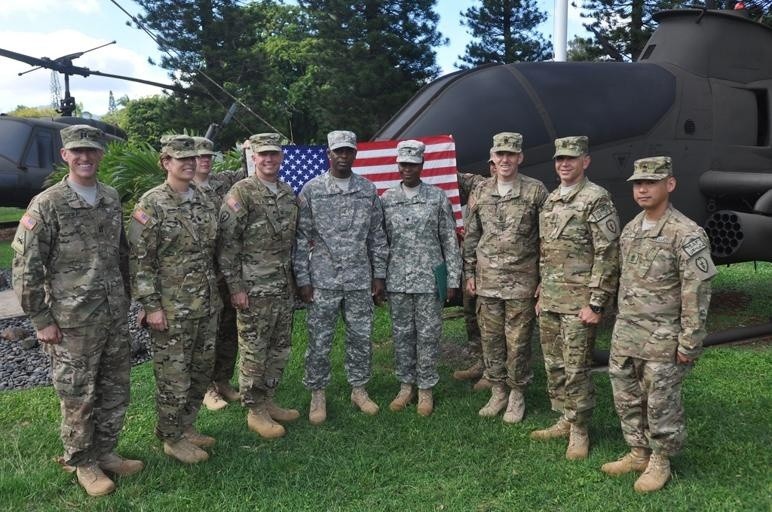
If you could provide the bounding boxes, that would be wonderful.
[390,357,673,493]
[351,388,380,415]
[216,380,241,402]
[202,384,228,411]
[96,450,145,477]
[76,462,116,498]
[308,389,327,424]
[184,425,215,447]
[247,404,285,439]
[163,438,209,465]
[265,397,300,422]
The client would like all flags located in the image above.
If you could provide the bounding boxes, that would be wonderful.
[275,133,465,248]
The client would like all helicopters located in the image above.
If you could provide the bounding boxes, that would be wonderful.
[0,40,238,229]
[367,0,772,368]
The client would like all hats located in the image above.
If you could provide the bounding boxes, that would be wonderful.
[492,132,523,154]
[327,130,357,150]
[192,137,217,157]
[396,140,425,163]
[487,148,493,164]
[626,156,675,184]
[59,124,108,155]
[552,134,589,160]
[249,133,282,153]
[160,135,201,159]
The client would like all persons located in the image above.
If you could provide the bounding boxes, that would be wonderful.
[216,133,301,441]
[379,139,462,419]
[529,133,623,460]
[292,129,391,426]
[126,133,225,465]
[598,152,721,494]
[10,125,146,497]
[185,136,254,414]
[462,131,549,423]
[451,147,498,394]
